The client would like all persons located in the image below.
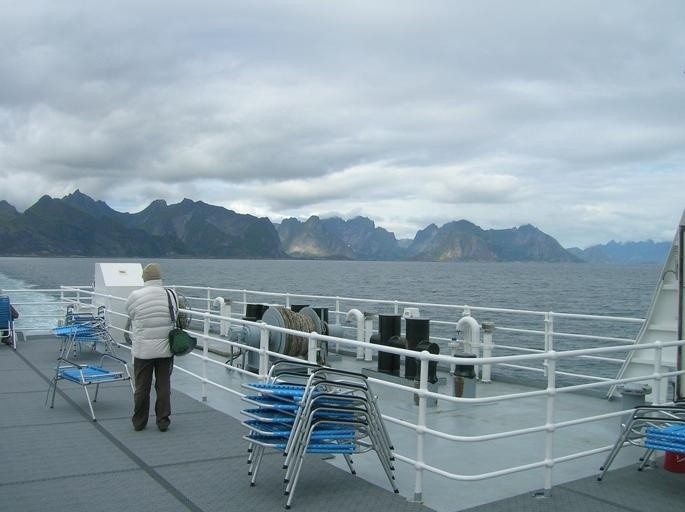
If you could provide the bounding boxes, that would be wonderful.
[123,263,179,432]
[2,304,19,345]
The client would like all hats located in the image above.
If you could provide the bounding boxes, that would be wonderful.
[142,262,161,282]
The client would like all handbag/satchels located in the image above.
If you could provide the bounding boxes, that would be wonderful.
[168,329,197,356]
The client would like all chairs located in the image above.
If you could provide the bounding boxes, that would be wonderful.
[0,295,18,351]
[594,397,685,483]
[238,359,400,509]
[43,354,134,422]
[52,303,119,362]
